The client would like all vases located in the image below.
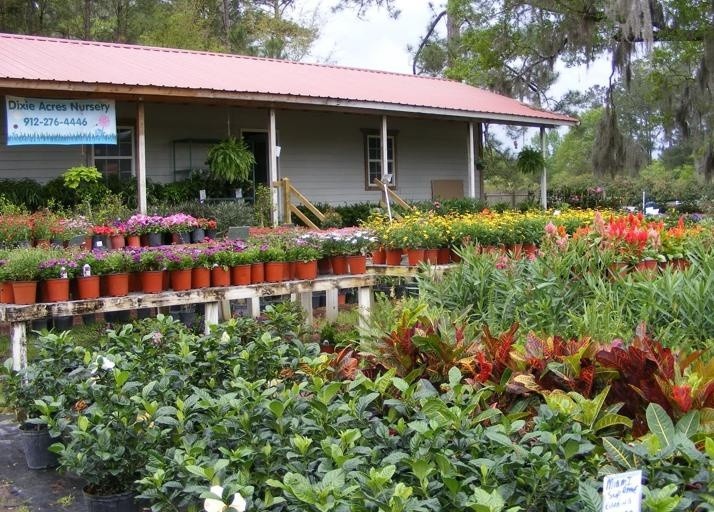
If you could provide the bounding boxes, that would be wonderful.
[0,262,266,306]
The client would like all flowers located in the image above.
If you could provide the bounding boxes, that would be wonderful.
[0,211,690,280]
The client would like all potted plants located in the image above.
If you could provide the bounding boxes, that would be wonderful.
[217,199,253,242]
[19,393,136,512]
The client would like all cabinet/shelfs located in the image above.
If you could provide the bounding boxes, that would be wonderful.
[172,138,224,183]
